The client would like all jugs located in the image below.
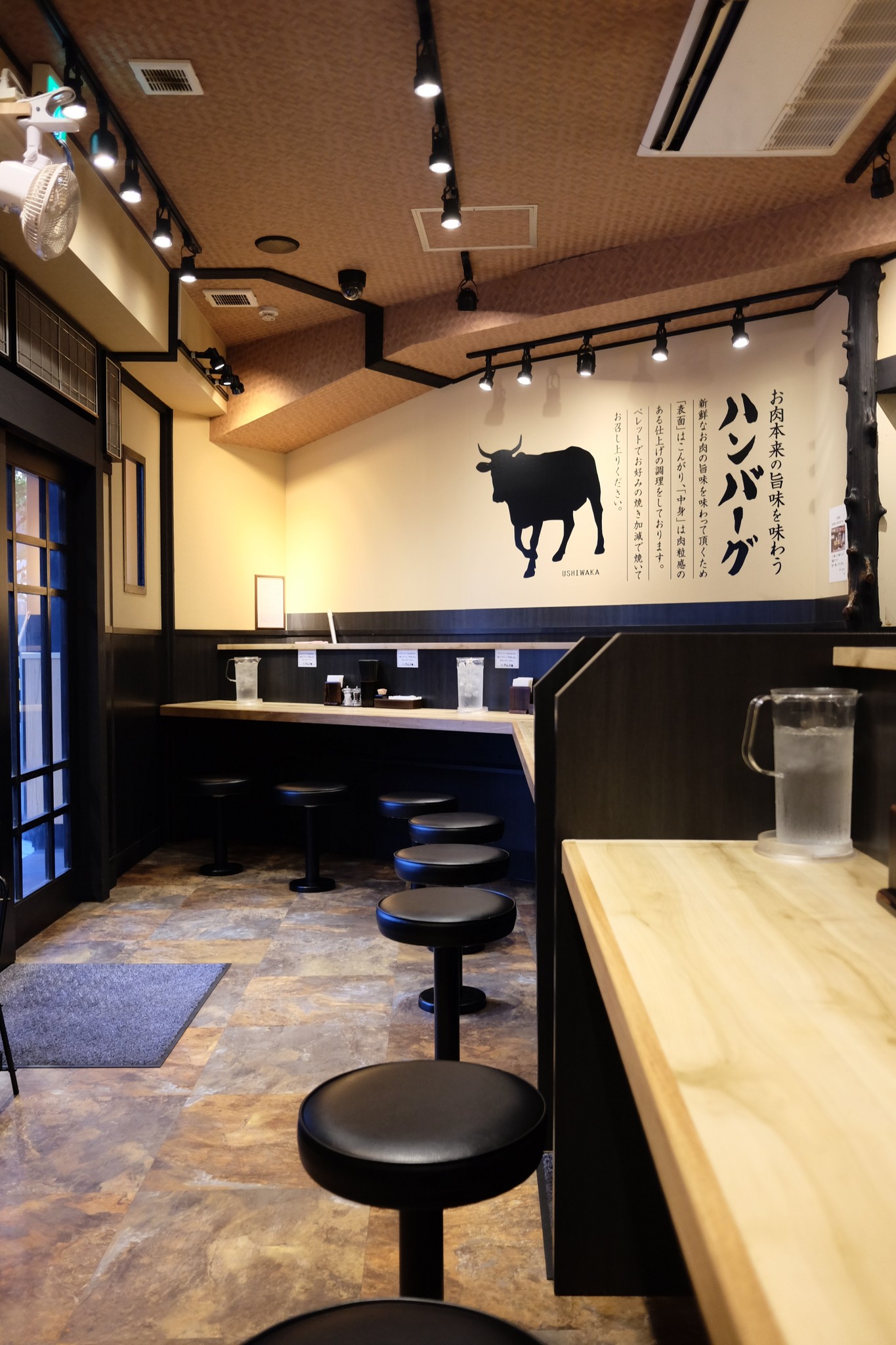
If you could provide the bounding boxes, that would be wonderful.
[456,656,484,709]
[742,686,863,845]
[225,656,262,701]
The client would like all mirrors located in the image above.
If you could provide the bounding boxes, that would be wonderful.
[122,444,146,595]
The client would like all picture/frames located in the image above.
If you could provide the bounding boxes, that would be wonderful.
[255,574,285,629]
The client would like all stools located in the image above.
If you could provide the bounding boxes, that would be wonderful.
[193,760,547,1345]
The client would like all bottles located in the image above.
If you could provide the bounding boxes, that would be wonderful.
[341,686,353,706]
[352,686,361,706]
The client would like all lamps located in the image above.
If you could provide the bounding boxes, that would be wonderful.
[179,239,197,282]
[441,183,462,229]
[414,41,441,97]
[189,348,245,395]
[515,302,750,384]
[89,97,120,169]
[455,277,479,311]
[119,150,142,202]
[62,44,88,118]
[871,148,896,198]
[429,122,452,172]
[152,201,173,248]
[476,355,496,390]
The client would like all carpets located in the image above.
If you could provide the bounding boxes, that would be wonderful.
[0,963,233,1068]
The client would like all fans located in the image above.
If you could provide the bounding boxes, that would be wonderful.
[0,67,82,259]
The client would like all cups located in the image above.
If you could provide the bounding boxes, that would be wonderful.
[361,681,378,708]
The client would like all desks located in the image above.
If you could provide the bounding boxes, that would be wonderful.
[542,629,896,1345]
[162,636,629,1280]
